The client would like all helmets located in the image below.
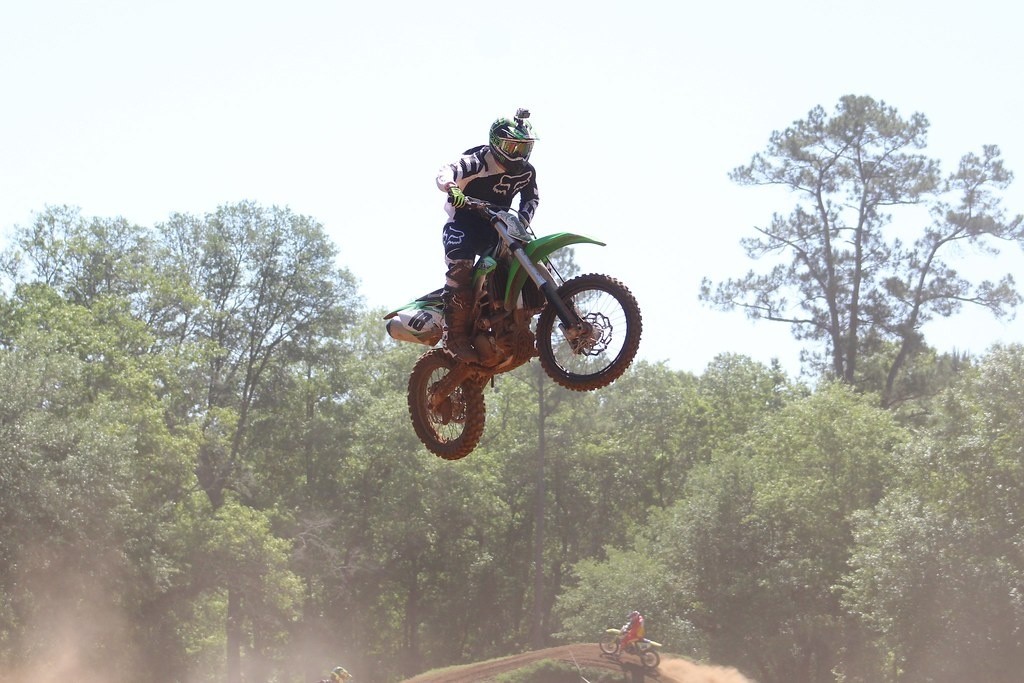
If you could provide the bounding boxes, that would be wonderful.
[629,611,640,621]
[489,117,540,175]
[330,667,352,683]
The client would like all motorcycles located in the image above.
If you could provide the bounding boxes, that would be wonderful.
[599,625,662,668]
[383,196,644,461]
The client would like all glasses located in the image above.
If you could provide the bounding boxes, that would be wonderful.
[493,137,535,162]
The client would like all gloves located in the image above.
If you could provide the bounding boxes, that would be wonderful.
[448,186,466,208]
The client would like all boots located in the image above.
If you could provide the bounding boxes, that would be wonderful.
[443,312,480,362]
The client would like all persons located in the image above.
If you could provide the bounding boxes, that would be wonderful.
[615,610,647,657]
[436,114,539,364]
[319,666,353,683]
